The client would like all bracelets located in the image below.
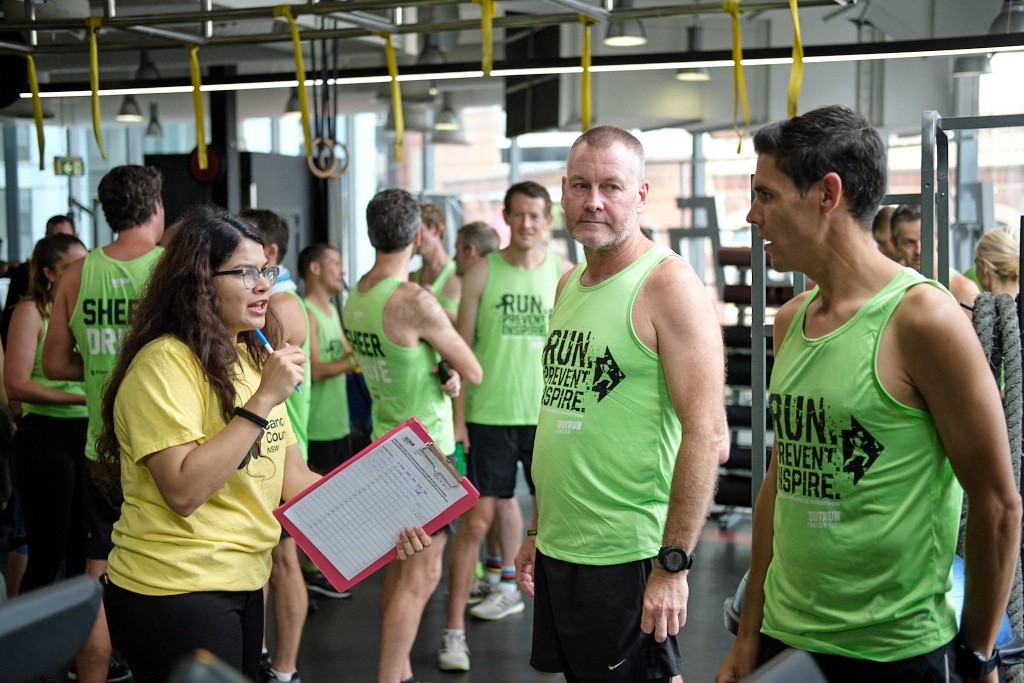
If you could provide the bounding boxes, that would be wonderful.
[528,529,537,536]
[348,354,355,373]
[234,407,268,428]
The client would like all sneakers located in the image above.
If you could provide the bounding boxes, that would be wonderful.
[437,630,471,671]
[444,576,500,605]
[469,588,525,621]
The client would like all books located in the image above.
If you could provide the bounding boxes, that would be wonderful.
[736,647,829,683]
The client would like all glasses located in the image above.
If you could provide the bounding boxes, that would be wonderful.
[213,266,280,289]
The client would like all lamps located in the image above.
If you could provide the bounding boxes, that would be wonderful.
[116,96,142,121]
[676,27,709,82]
[286,88,300,114]
[147,103,161,135]
[953,54,991,76]
[604,1,645,48]
[989,0,1024,34]
[417,33,446,63]
[434,90,459,130]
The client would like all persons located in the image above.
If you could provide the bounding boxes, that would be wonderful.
[4,232,89,593]
[972,227,1024,404]
[408,204,462,321]
[91,198,446,683]
[437,181,577,667]
[452,222,525,619]
[344,189,483,683]
[0,215,79,353]
[41,164,165,683]
[715,105,1022,683]
[233,209,312,683]
[514,124,730,683]
[298,245,360,614]
[890,204,981,321]
[873,206,905,266]
[0,237,19,278]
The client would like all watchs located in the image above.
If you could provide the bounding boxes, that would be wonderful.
[954,632,1001,681]
[657,544,695,573]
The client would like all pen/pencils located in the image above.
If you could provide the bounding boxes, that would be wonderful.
[255,328,302,395]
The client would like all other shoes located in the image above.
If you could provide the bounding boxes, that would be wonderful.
[267,666,303,683]
[66,654,132,682]
[301,563,353,599]
